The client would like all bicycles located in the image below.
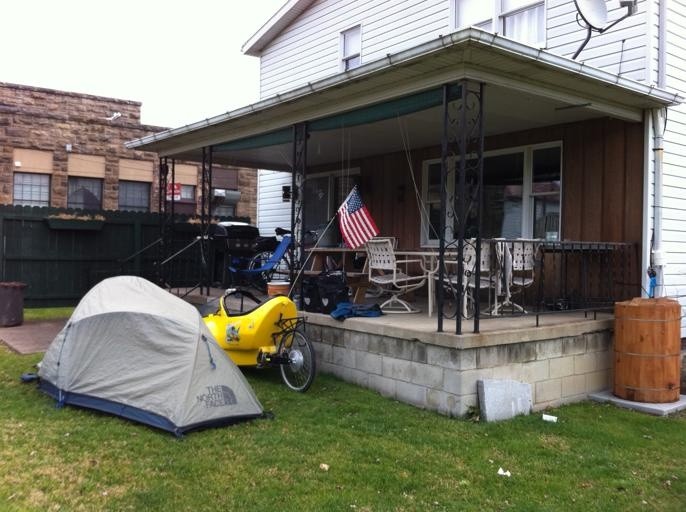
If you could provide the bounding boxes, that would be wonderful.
[245,222,301,288]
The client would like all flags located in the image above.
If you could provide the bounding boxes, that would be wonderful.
[334,186,380,252]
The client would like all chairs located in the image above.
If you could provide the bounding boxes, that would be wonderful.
[480,238,546,316]
[365,239,426,313]
[428,238,507,319]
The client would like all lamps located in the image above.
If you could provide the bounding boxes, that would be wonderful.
[283,186,292,201]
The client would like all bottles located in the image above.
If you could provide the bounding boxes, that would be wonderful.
[292,287,302,311]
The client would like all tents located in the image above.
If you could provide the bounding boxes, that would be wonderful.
[18,274,267,439]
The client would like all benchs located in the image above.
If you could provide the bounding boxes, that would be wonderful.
[276,269,367,287]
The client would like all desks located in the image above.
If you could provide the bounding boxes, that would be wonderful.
[395,251,458,317]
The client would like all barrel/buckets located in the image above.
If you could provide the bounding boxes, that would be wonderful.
[266,282,292,300]
[613,296,683,404]
